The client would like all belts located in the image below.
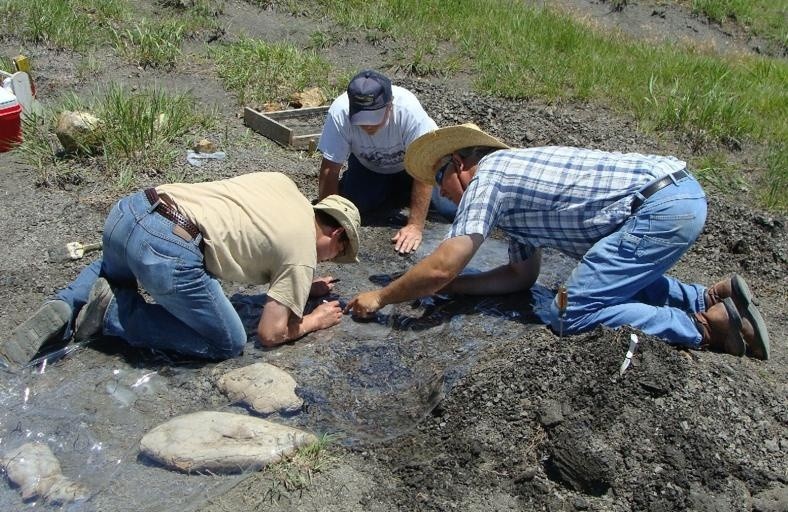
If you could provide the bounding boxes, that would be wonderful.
[630,168,687,208]
[143,186,207,255]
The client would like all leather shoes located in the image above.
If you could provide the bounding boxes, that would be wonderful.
[0,300,75,370]
[691,296,746,358]
[73,273,113,340]
[702,273,774,361]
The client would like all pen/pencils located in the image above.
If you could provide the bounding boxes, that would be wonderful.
[329,278,342,283]
[322,299,328,303]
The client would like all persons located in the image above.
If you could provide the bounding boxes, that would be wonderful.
[313,69,460,258]
[1,169,364,368]
[342,124,772,362]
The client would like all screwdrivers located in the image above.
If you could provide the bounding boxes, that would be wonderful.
[558,285,567,346]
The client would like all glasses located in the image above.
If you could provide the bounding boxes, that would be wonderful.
[340,241,347,259]
[434,160,450,184]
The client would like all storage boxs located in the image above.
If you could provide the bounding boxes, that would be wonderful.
[0,87,22,152]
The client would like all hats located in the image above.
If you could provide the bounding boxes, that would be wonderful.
[346,69,394,128]
[313,193,363,265]
[404,121,508,184]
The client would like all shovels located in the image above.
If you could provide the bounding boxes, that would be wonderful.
[47,239,104,263]
